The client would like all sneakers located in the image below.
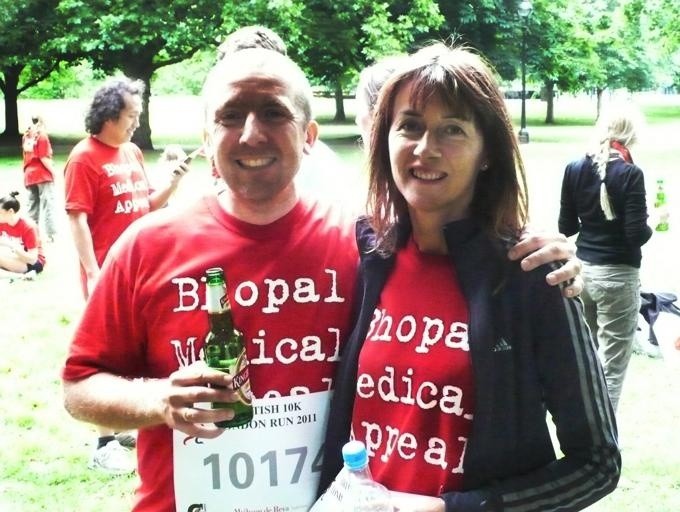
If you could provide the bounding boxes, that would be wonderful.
[113,429,142,451]
[85,439,136,478]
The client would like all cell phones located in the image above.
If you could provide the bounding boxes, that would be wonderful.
[174,157,192,175]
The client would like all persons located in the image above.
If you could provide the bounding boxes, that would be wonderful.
[23,115,59,245]
[557,82,653,428]
[212,19,369,197]
[58,48,590,511]
[0,191,46,278]
[60,75,208,473]
[301,39,624,511]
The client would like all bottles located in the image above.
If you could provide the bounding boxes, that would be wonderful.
[340,440,394,512]
[202,268,254,429]
